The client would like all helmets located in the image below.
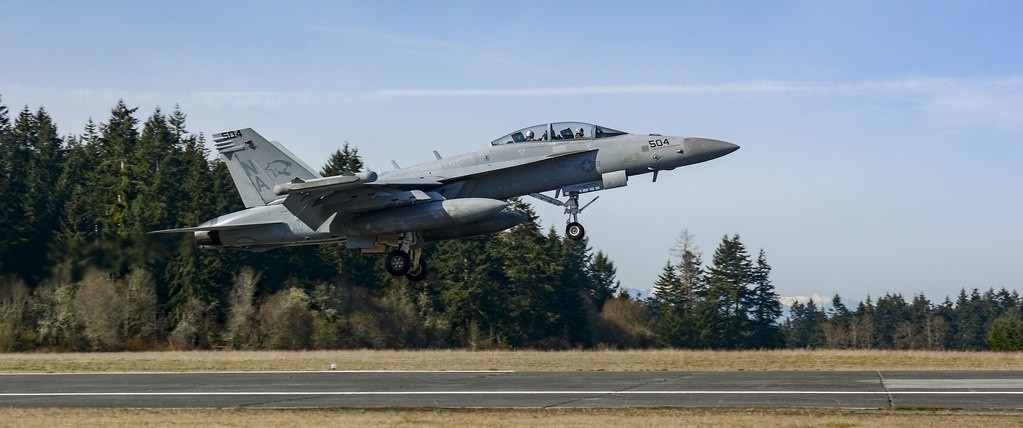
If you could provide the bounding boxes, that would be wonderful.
[576,127,584,135]
[526,130,534,138]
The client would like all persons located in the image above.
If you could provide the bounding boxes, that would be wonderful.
[575,127,585,139]
[523,130,534,141]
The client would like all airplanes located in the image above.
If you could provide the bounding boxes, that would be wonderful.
[144,121,741,282]
